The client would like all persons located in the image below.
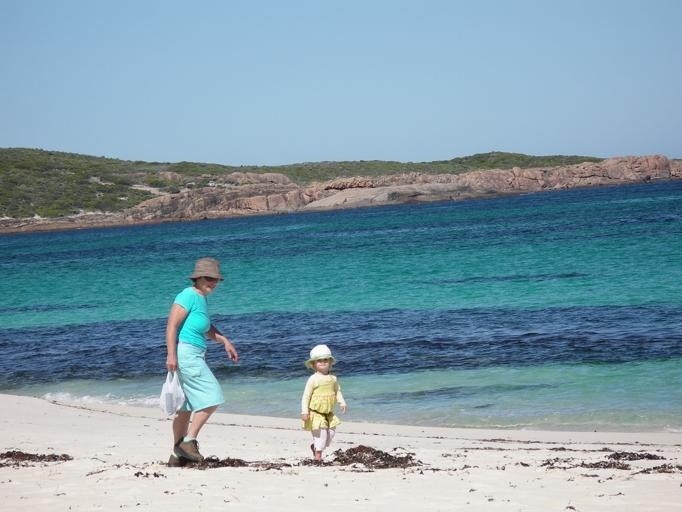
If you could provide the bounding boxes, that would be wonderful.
[165,257,238,467]
[300,345,347,462]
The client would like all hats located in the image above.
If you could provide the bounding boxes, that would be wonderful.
[189,258,222,281]
[304,345,337,367]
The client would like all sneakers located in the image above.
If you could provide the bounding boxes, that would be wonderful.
[169,438,205,466]
